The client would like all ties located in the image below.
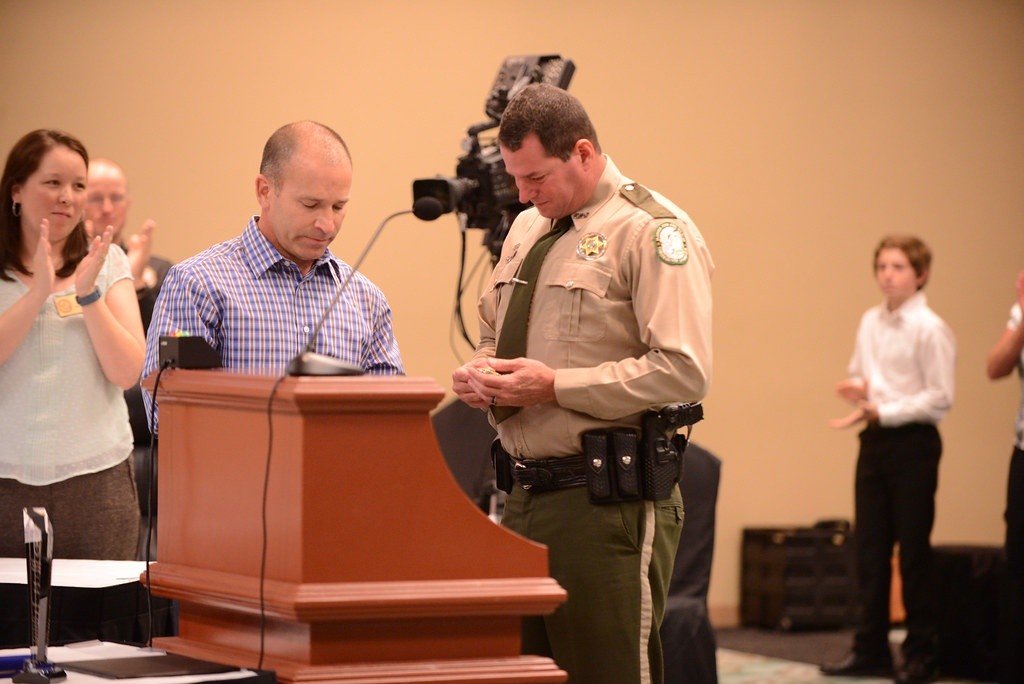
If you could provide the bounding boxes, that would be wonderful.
[489,215,572,424]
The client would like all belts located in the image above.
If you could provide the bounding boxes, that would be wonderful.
[506,455,587,494]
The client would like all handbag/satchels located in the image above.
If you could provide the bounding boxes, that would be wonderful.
[739,520,860,630]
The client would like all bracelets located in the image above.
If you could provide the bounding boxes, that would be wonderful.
[75,285,101,306]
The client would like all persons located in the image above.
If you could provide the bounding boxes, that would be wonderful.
[451,83,715,684]
[986,273,1024,684]
[82,155,174,522]
[818,236,957,684]
[0,129,148,560]
[139,121,407,435]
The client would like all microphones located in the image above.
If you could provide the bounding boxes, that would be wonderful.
[285,197,443,376]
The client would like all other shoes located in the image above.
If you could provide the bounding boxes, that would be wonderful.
[819,649,894,677]
[895,653,942,684]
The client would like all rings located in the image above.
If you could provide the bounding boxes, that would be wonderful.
[490,396,497,405]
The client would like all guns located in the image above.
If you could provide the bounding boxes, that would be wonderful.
[644,397,705,466]
